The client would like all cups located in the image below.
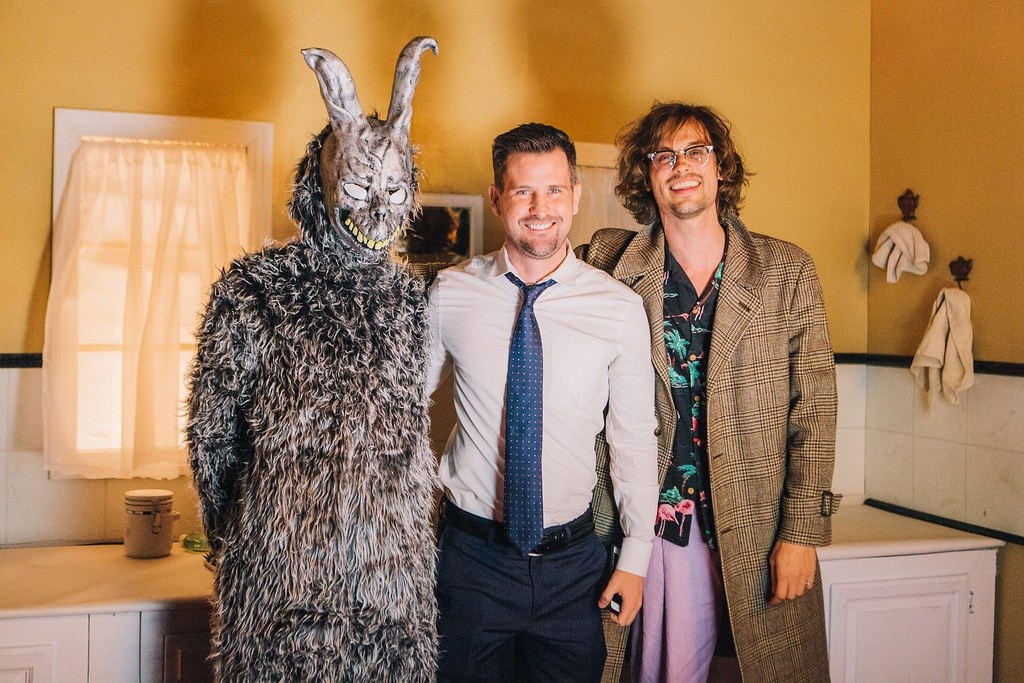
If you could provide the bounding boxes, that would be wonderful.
[122,489,181,558]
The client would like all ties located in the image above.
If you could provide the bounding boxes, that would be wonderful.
[504,272,557,556]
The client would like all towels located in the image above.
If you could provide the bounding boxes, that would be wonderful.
[871,220,932,284]
[908,285,976,417]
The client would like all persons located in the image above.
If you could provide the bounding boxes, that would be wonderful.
[427,123,660,683]
[410,102,837,683]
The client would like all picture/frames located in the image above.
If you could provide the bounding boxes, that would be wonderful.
[395,193,484,283]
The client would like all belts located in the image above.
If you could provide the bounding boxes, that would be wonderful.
[439,498,595,554]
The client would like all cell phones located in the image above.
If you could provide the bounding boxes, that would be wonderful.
[609,546,622,615]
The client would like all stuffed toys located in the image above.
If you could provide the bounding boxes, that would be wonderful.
[187,34,438,683]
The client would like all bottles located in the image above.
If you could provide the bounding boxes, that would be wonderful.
[178,533,209,551]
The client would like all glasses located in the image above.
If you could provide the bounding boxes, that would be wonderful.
[647,145,713,170]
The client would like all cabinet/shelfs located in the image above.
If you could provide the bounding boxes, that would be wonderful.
[821,575,970,683]
[0,608,211,683]
